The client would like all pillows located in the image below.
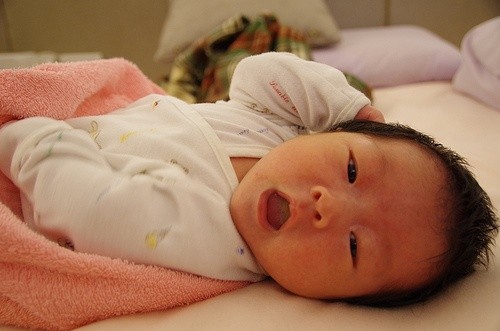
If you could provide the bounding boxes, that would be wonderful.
[311,24,463,87]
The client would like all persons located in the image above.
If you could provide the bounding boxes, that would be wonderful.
[0,51,496,308]
